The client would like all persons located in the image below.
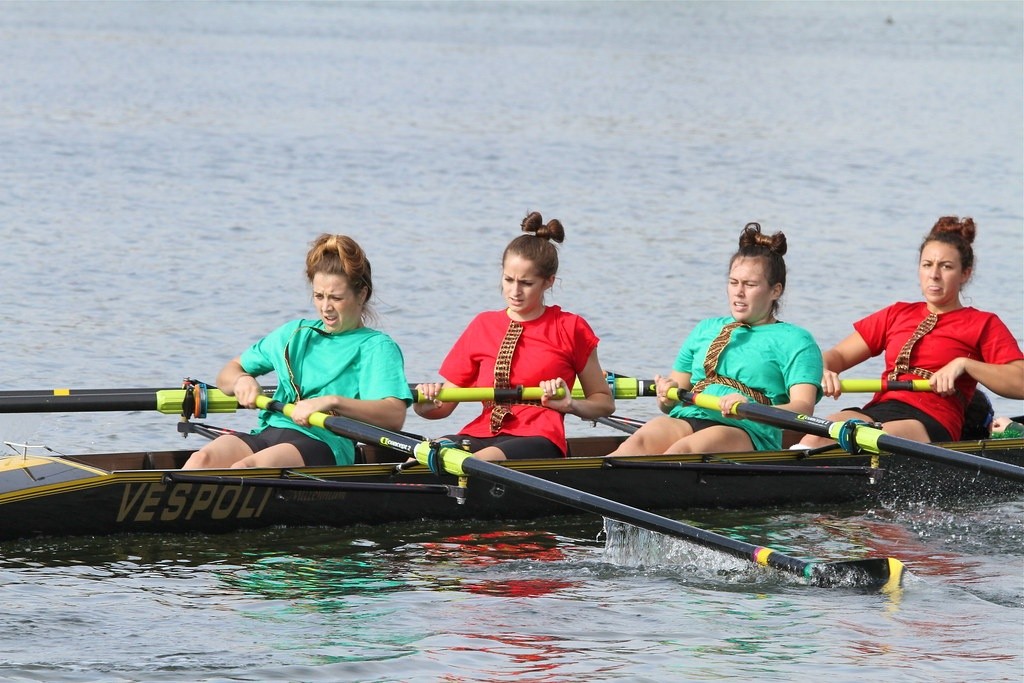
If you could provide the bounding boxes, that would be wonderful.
[405,213,615,464]
[605,222,823,458]
[182,233,414,470]
[792,215,1024,450]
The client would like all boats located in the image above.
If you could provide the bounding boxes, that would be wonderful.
[0,412,1024,537]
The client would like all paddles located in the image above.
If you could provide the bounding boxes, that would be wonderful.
[256,394,910,593]
[0,371,568,419]
[666,386,1024,483]
[570,378,935,399]
